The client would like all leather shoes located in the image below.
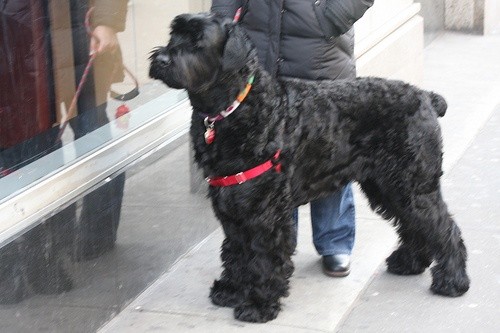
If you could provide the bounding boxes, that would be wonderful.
[322,253,351,276]
[74,239,115,262]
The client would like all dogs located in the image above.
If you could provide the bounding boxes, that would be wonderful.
[0,13,73,305]
[146,11,470,324]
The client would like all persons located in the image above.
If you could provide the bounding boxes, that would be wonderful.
[0,0,130,259]
[209,0,375,277]
